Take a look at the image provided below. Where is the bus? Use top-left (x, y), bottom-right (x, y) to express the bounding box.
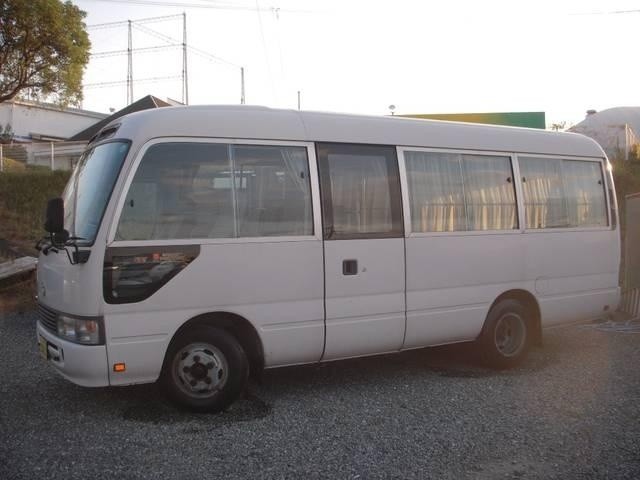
top-left (32, 103), bottom-right (625, 413)
